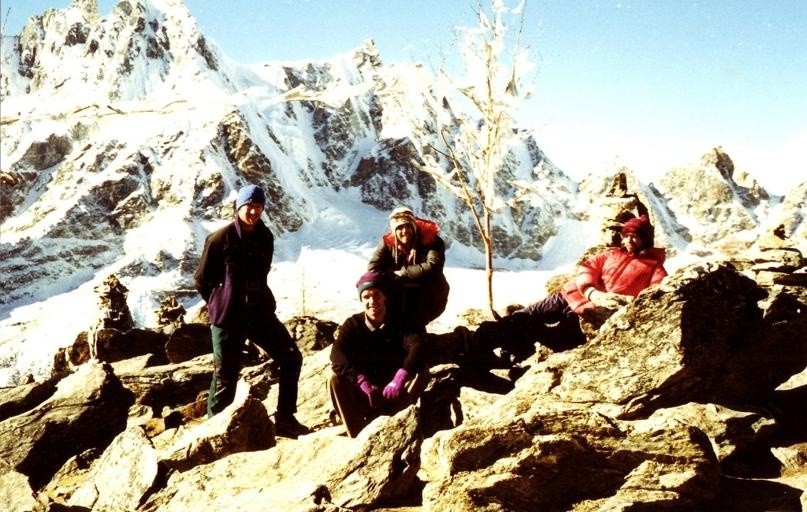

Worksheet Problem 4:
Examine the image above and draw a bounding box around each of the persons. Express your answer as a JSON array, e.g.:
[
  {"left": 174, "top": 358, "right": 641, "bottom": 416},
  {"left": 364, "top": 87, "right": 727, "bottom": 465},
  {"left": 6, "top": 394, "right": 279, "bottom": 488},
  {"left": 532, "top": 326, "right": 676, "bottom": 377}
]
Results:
[
  {"left": 367, "top": 204, "right": 451, "bottom": 333},
  {"left": 192, "top": 182, "right": 310, "bottom": 437},
  {"left": 328, "top": 273, "right": 429, "bottom": 437},
  {"left": 454, "top": 213, "right": 669, "bottom": 364}
]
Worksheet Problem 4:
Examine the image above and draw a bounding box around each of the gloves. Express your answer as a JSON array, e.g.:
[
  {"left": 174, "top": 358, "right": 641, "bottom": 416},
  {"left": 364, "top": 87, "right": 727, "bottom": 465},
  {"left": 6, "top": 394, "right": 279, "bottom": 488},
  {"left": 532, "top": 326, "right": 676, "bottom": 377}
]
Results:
[
  {"left": 589, "top": 289, "right": 636, "bottom": 312},
  {"left": 357, "top": 374, "right": 378, "bottom": 407},
  {"left": 382, "top": 368, "right": 408, "bottom": 399}
]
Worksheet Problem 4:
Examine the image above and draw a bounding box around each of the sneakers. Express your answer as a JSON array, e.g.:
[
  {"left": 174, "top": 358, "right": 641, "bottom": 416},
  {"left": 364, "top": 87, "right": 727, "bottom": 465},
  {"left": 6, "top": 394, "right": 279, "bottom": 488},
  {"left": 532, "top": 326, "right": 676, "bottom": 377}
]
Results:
[{"left": 274, "top": 412, "right": 308, "bottom": 432}]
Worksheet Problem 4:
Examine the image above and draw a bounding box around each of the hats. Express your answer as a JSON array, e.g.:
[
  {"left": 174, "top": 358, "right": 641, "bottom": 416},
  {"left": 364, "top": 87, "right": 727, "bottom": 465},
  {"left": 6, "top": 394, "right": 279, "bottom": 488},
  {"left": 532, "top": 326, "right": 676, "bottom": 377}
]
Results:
[
  {"left": 389, "top": 206, "right": 417, "bottom": 236},
  {"left": 622, "top": 214, "right": 649, "bottom": 238},
  {"left": 236, "top": 184, "right": 265, "bottom": 211},
  {"left": 356, "top": 271, "right": 379, "bottom": 301}
]
